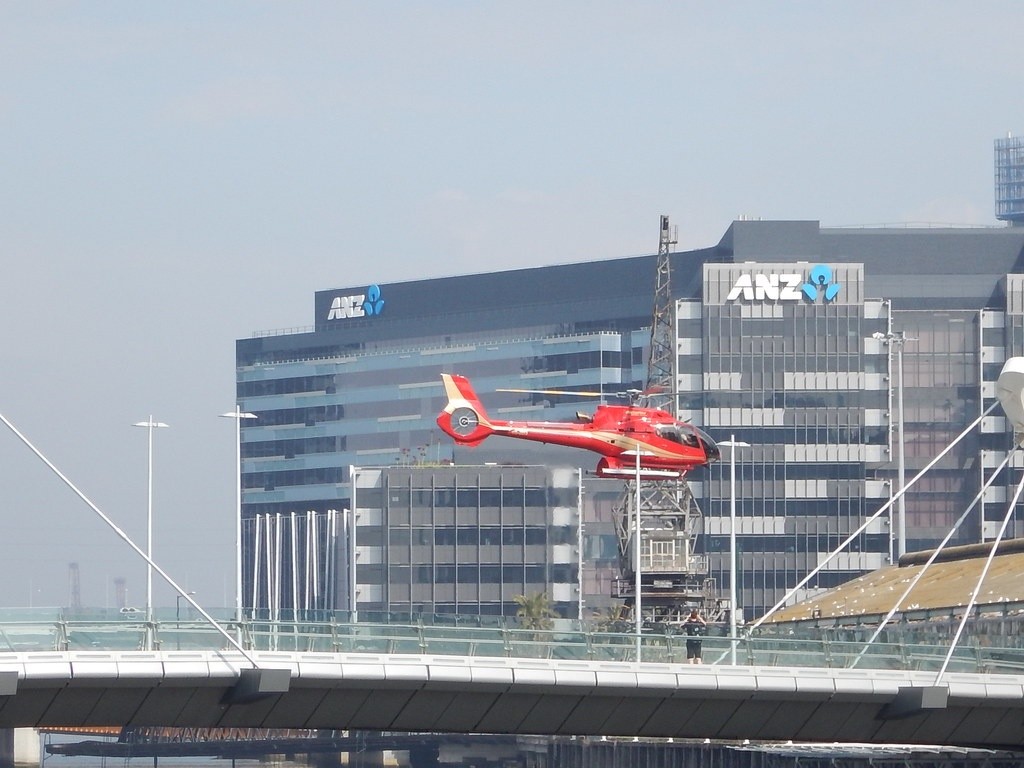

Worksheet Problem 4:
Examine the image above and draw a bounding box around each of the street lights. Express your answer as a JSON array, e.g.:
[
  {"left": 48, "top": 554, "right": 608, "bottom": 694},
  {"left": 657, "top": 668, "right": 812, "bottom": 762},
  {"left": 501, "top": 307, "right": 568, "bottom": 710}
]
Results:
[
  {"left": 620, "top": 444, "right": 654, "bottom": 664},
  {"left": 177, "top": 591, "right": 197, "bottom": 651},
  {"left": 132, "top": 414, "right": 170, "bottom": 650},
  {"left": 218, "top": 405, "right": 258, "bottom": 651},
  {"left": 714, "top": 434, "right": 751, "bottom": 665}
]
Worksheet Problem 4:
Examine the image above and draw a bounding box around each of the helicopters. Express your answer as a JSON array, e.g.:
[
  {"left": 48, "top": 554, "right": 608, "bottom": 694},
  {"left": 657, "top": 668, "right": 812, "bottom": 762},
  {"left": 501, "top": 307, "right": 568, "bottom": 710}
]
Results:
[{"left": 437, "top": 373, "right": 720, "bottom": 480}]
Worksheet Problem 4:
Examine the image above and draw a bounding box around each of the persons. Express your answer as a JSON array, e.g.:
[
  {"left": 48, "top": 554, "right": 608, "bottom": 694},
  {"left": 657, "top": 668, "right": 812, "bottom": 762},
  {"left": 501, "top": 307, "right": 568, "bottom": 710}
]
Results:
[
  {"left": 680, "top": 430, "right": 697, "bottom": 446},
  {"left": 681, "top": 610, "right": 708, "bottom": 664}
]
